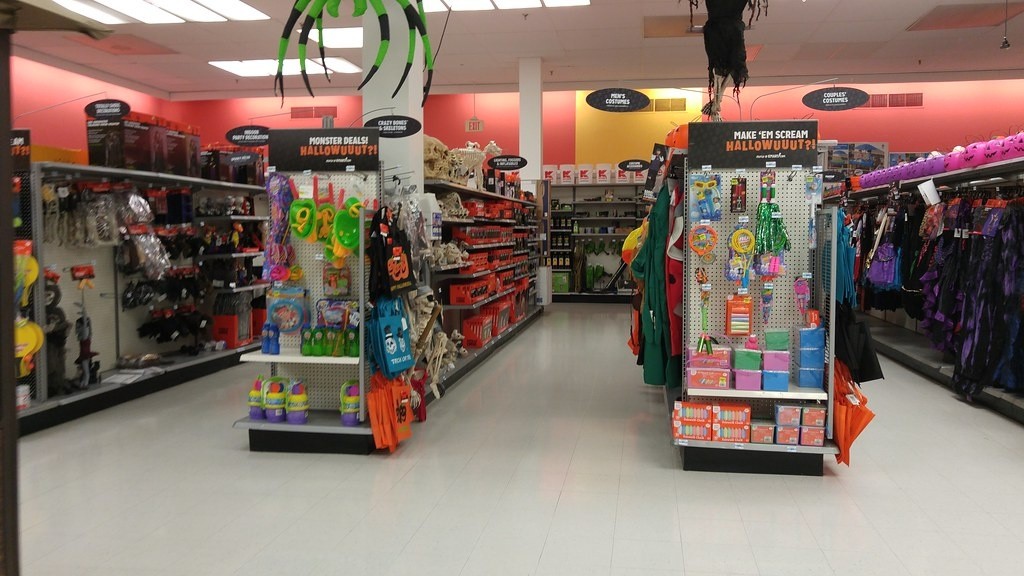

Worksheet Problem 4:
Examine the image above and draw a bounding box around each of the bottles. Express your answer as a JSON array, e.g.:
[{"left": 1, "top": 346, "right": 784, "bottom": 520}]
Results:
[
  {"left": 557, "top": 233, "right": 563, "bottom": 249},
  {"left": 559, "top": 252, "right": 564, "bottom": 269},
  {"left": 551, "top": 233, "right": 557, "bottom": 249},
  {"left": 553, "top": 252, "right": 557, "bottom": 268},
  {"left": 542, "top": 251, "right": 549, "bottom": 266},
  {"left": 555, "top": 212, "right": 560, "bottom": 229},
  {"left": 573, "top": 221, "right": 578, "bottom": 234},
  {"left": 564, "top": 233, "right": 570, "bottom": 249},
  {"left": 565, "top": 252, "right": 570, "bottom": 268},
  {"left": 550, "top": 216, "right": 555, "bottom": 229},
  {"left": 561, "top": 212, "right": 565, "bottom": 229},
  {"left": 567, "top": 212, "right": 572, "bottom": 229}
]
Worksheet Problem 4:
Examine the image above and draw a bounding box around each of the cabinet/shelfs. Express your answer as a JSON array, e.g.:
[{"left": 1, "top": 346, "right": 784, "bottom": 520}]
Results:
[{"left": 0, "top": 120, "right": 1024, "bottom": 477}]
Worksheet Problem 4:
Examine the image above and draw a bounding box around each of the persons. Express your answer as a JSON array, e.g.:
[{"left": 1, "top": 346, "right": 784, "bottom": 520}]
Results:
[
  {"left": 258, "top": 157, "right": 264, "bottom": 185},
  {"left": 154, "top": 132, "right": 170, "bottom": 173},
  {"left": 189, "top": 140, "right": 199, "bottom": 175},
  {"left": 104, "top": 131, "right": 121, "bottom": 166}
]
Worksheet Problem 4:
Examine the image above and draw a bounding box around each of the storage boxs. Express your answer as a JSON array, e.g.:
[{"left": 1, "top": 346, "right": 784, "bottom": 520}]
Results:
[
  {"left": 200, "top": 140, "right": 270, "bottom": 186},
  {"left": 462, "top": 314, "right": 494, "bottom": 348},
  {"left": 459, "top": 252, "right": 490, "bottom": 275},
  {"left": 552, "top": 273, "right": 572, "bottom": 293},
  {"left": 84, "top": 98, "right": 203, "bottom": 177},
  {"left": 450, "top": 280, "right": 489, "bottom": 306},
  {"left": 452, "top": 226, "right": 486, "bottom": 245},
  {"left": 481, "top": 301, "right": 512, "bottom": 337},
  {"left": 529, "top": 265, "right": 552, "bottom": 306}
]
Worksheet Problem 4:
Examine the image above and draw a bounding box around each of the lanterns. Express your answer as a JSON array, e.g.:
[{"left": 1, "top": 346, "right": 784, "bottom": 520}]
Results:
[{"left": 859, "top": 132, "right": 1024, "bottom": 189}]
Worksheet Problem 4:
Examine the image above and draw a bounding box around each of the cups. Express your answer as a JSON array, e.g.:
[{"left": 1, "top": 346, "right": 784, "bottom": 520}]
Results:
[
  {"left": 586, "top": 228, "right": 593, "bottom": 234},
  {"left": 600, "top": 227, "right": 607, "bottom": 234},
  {"left": 595, "top": 228, "right": 600, "bottom": 234},
  {"left": 608, "top": 227, "right": 614, "bottom": 233},
  {"left": 579, "top": 227, "right": 585, "bottom": 233}
]
